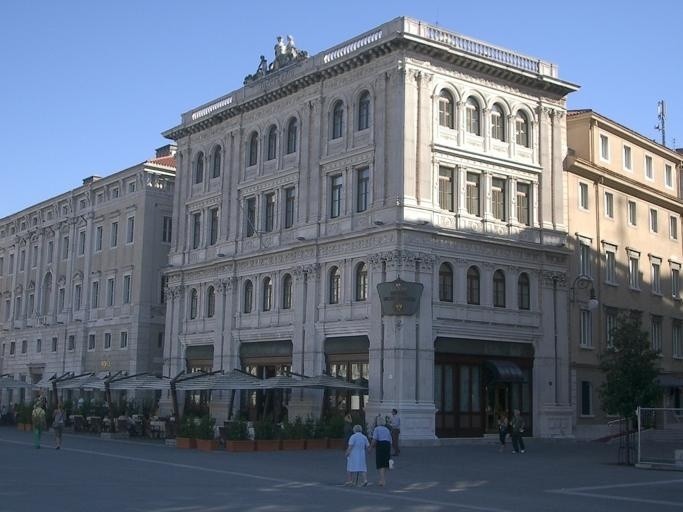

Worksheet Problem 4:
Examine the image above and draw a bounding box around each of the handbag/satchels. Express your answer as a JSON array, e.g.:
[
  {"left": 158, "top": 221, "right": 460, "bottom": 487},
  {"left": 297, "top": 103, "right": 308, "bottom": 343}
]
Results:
[
  {"left": 51, "top": 421, "right": 59, "bottom": 428},
  {"left": 388, "top": 460, "right": 393, "bottom": 470}
]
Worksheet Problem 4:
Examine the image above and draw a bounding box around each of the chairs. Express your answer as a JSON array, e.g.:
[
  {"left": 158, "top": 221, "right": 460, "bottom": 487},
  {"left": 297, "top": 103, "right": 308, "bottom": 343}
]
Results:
[{"left": 69, "top": 413, "right": 172, "bottom": 439}]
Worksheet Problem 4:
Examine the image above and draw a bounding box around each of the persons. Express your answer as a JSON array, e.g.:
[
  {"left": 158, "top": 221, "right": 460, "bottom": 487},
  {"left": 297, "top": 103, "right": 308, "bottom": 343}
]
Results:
[
  {"left": 386, "top": 408, "right": 401, "bottom": 456},
  {"left": 256, "top": 55, "right": 267, "bottom": 76},
  {"left": 507, "top": 409, "right": 527, "bottom": 453},
  {"left": 495, "top": 411, "right": 509, "bottom": 449},
  {"left": 366, "top": 415, "right": 392, "bottom": 485},
  {"left": 343, "top": 424, "right": 370, "bottom": 486},
  {"left": 30, "top": 400, "right": 45, "bottom": 449},
  {"left": 52, "top": 401, "right": 64, "bottom": 449},
  {"left": 343, "top": 413, "right": 352, "bottom": 448},
  {"left": 484, "top": 400, "right": 510, "bottom": 433},
  {"left": 273, "top": 36, "right": 285, "bottom": 57},
  {"left": 285, "top": 34, "right": 296, "bottom": 60}
]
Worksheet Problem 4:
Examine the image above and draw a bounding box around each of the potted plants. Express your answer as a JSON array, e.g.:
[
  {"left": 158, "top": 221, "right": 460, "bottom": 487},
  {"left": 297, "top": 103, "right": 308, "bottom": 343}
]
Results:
[
  {"left": 16, "top": 402, "right": 34, "bottom": 433},
  {"left": 172, "top": 413, "right": 350, "bottom": 452}
]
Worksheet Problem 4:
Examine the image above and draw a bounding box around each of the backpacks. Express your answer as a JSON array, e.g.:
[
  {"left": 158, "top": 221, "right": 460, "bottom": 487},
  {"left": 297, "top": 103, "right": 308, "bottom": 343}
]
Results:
[{"left": 32, "top": 409, "right": 42, "bottom": 427}]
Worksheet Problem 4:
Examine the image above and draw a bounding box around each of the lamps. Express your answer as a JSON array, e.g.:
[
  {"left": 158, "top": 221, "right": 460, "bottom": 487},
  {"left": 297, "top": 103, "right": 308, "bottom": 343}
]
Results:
[{"left": 570, "top": 273, "right": 599, "bottom": 310}]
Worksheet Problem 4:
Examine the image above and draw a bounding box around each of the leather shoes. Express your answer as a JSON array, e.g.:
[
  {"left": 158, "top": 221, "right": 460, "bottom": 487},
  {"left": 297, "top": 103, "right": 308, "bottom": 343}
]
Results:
[
  {"left": 344, "top": 481, "right": 352, "bottom": 486},
  {"left": 360, "top": 480, "right": 367, "bottom": 486}
]
